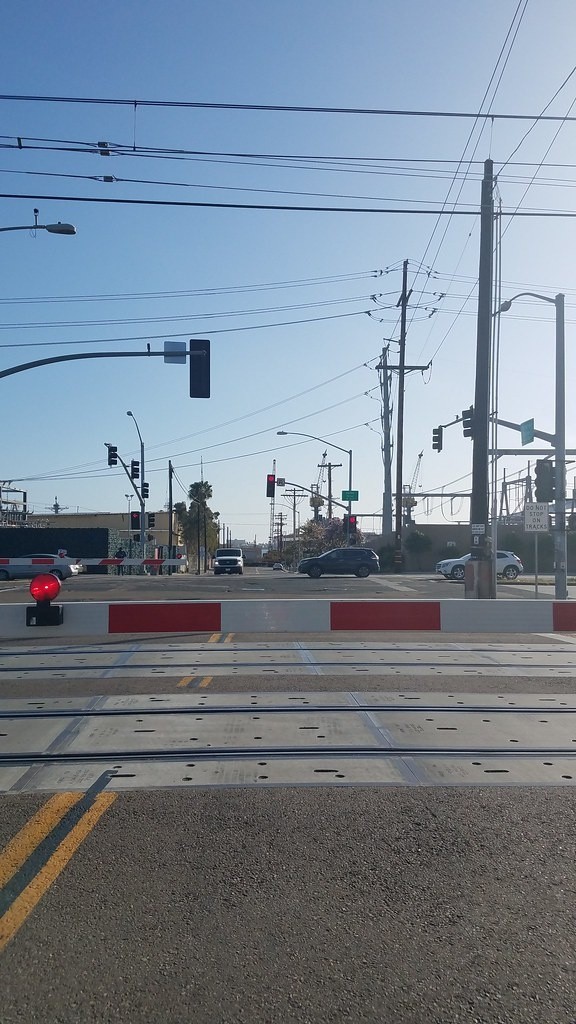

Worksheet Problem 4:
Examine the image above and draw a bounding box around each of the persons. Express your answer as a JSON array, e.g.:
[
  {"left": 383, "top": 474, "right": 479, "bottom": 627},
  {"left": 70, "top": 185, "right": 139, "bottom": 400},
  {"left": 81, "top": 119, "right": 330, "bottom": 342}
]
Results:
[{"left": 114, "top": 547, "right": 127, "bottom": 576}]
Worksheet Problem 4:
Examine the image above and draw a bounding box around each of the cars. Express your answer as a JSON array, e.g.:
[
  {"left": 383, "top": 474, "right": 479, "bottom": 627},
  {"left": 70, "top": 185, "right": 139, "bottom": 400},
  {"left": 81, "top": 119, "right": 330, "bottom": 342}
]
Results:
[
  {"left": 0, "top": 554, "right": 84, "bottom": 581},
  {"left": 298, "top": 547, "right": 380, "bottom": 578},
  {"left": 211, "top": 548, "right": 246, "bottom": 575},
  {"left": 273, "top": 562, "right": 283, "bottom": 570},
  {"left": 436, "top": 550, "right": 523, "bottom": 580}
]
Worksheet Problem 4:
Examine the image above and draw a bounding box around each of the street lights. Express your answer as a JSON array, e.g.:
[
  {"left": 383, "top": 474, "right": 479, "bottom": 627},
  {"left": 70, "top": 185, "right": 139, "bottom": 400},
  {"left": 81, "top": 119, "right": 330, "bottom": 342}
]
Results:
[
  {"left": 498, "top": 293, "right": 569, "bottom": 601},
  {"left": 277, "top": 431, "right": 352, "bottom": 547},
  {"left": 127, "top": 411, "right": 150, "bottom": 575},
  {"left": 125, "top": 494, "right": 134, "bottom": 575},
  {"left": 270, "top": 503, "right": 300, "bottom": 563}
]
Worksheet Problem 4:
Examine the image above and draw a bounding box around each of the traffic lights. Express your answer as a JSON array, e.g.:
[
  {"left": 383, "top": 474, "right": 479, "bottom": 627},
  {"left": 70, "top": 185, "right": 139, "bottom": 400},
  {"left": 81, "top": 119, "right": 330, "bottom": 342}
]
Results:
[
  {"left": 108, "top": 446, "right": 118, "bottom": 465},
  {"left": 535, "top": 459, "right": 556, "bottom": 503},
  {"left": 131, "top": 511, "right": 140, "bottom": 530},
  {"left": 134, "top": 534, "right": 154, "bottom": 542},
  {"left": 148, "top": 514, "right": 155, "bottom": 528},
  {"left": 267, "top": 474, "right": 275, "bottom": 497},
  {"left": 349, "top": 516, "right": 356, "bottom": 533},
  {"left": 142, "top": 483, "right": 149, "bottom": 498},
  {"left": 131, "top": 461, "right": 139, "bottom": 479},
  {"left": 432, "top": 405, "right": 473, "bottom": 453}
]
